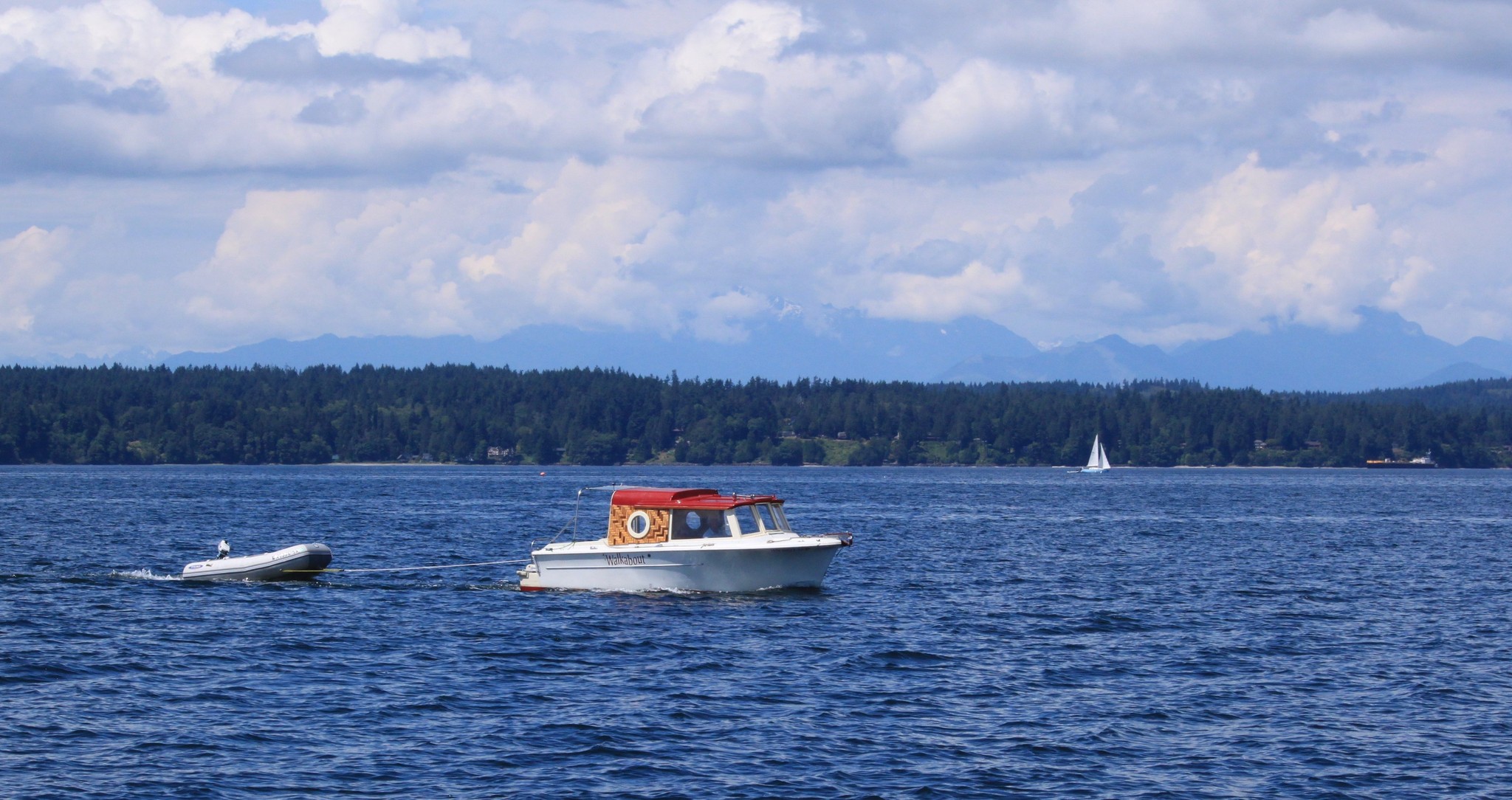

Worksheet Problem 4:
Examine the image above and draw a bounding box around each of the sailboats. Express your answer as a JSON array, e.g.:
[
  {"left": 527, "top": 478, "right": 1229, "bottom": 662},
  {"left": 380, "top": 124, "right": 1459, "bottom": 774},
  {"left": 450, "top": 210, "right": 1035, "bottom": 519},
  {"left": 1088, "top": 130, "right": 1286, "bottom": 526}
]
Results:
[{"left": 1075, "top": 433, "right": 1112, "bottom": 474}]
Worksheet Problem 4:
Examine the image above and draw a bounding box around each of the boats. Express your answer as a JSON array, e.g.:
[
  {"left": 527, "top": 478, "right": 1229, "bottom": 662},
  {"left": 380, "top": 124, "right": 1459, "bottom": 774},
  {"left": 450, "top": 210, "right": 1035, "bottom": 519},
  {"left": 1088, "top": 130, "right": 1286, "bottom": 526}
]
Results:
[
  {"left": 517, "top": 483, "right": 853, "bottom": 597},
  {"left": 1365, "top": 448, "right": 1438, "bottom": 469},
  {"left": 181, "top": 539, "right": 333, "bottom": 582}
]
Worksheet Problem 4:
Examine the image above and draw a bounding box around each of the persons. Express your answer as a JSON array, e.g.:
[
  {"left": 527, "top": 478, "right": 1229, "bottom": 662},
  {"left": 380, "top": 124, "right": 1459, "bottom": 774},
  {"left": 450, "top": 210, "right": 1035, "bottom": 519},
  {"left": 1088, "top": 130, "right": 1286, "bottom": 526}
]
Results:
[{"left": 703, "top": 513, "right": 733, "bottom": 538}]
